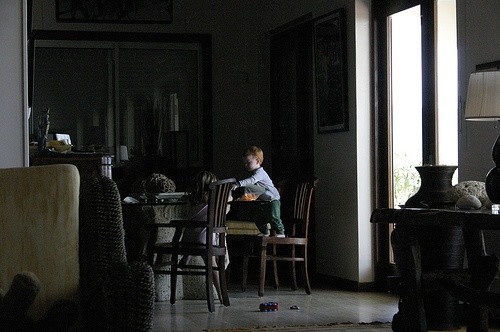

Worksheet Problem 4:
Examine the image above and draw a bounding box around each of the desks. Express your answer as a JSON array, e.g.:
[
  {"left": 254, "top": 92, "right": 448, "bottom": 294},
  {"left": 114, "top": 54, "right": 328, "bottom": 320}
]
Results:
[
  {"left": 123, "top": 200, "right": 276, "bottom": 297},
  {"left": 369, "top": 207, "right": 500, "bottom": 332}
]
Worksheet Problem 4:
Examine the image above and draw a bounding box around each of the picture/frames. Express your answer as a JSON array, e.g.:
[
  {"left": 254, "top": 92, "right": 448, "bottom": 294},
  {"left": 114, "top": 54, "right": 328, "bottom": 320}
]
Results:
[
  {"left": 312, "top": 6, "right": 350, "bottom": 134},
  {"left": 55, "top": 0, "right": 173, "bottom": 24}
]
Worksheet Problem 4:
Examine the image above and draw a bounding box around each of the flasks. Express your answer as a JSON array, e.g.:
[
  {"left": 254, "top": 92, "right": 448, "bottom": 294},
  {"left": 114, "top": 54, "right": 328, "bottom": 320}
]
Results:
[{"left": 101, "top": 157, "right": 113, "bottom": 180}]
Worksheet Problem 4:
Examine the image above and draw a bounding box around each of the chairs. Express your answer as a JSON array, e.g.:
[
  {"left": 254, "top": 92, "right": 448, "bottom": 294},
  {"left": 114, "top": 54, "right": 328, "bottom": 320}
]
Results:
[
  {"left": 241, "top": 176, "right": 321, "bottom": 296},
  {"left": 143, "top": 177, "right": 237, "bottom": 313}
]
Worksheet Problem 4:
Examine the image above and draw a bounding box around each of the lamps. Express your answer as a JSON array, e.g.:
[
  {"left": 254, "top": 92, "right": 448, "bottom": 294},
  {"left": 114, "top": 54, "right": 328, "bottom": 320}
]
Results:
[{"left": 462, "top": 60, "right": 500, "bottom": 211}]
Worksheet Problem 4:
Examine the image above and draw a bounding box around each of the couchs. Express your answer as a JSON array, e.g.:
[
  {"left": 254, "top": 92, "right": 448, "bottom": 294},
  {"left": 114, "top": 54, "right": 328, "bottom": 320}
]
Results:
[{"left": 0, "top": 163, "right": 156, "bottom": 332}]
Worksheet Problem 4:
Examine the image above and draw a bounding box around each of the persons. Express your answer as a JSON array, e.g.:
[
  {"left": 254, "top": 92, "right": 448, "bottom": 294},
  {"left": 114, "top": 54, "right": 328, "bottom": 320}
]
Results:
[
  {"left": 230, "top": 146, "right": 285, "bottom": 237},
  {"left": 179, "top": 171, "right": 230, "bottom": 304}
]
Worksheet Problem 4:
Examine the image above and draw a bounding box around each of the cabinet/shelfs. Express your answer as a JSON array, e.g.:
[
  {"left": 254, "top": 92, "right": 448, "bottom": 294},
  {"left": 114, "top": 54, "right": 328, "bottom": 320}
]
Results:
[{"left": 35, "top": 153, "right": 165, "bottom": 197}]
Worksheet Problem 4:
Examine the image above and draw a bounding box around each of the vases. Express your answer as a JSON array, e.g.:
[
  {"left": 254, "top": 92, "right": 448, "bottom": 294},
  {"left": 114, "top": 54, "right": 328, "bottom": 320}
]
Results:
[{"left": 390, "top": 164, "right": 465, "bottom": 332}]
